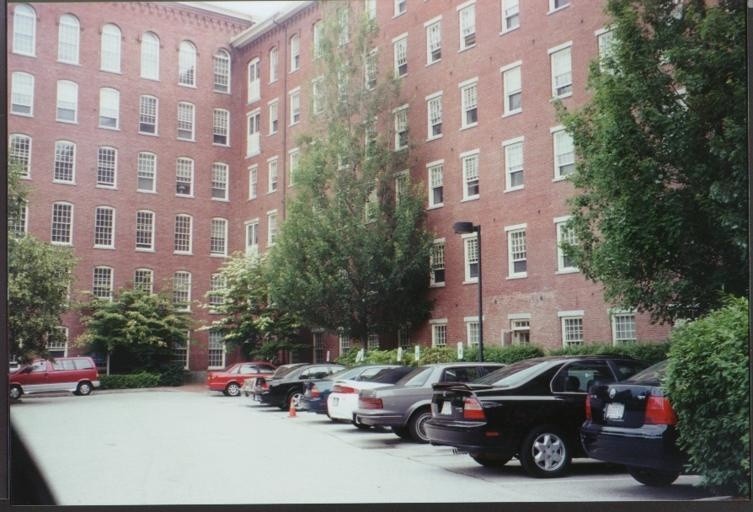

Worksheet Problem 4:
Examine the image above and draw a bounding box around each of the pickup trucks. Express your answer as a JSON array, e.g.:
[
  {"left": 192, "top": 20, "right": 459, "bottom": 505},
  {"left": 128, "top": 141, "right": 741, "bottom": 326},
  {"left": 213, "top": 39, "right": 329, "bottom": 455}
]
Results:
[{"left": 240, "top": 364, "right": 308, "bottom": 404}]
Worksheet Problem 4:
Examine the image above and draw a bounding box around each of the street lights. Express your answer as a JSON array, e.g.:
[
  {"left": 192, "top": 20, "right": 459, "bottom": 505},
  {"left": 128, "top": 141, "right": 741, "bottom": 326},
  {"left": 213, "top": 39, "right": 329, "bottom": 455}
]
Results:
[{"left": 454, "top": 222, "right": 484, "bottom": 362}]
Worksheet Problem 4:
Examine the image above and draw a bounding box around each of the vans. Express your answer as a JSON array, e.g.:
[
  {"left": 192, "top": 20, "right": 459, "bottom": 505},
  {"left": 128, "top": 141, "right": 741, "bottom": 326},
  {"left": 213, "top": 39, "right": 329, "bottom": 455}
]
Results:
[{"left": 7, "top": 356, "right": 101, "bottom": 399}]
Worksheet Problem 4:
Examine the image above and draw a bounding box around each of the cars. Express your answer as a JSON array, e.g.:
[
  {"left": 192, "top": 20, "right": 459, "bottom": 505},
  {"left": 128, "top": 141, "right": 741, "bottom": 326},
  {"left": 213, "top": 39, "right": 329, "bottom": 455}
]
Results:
[
  {"left": 423, "top": 354, "right": 655, "bottom": 479},
  {"left": 579, "top": 357, "right": 706, "bottom": 487},
  {"left": 357, "top": 362, "right": 510, "bottom": 444},
  {"left": 300, "top": 364, "right": 375, "bottom": 419},
  {"left": 206, "top": 362, "right": 277, "bottom": 398},
  {"left": 253, "top": 363, "right": 347, "bottom": 412},
  {"left": 326, "top": 365, "right": 424, "bottom": 433}
]
